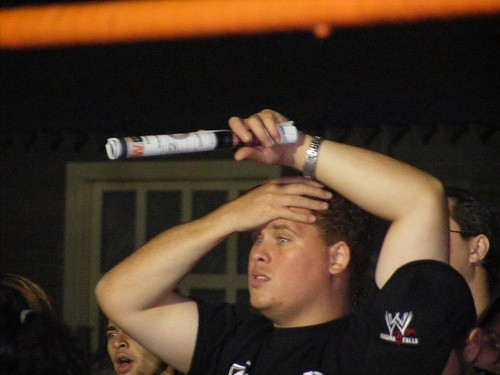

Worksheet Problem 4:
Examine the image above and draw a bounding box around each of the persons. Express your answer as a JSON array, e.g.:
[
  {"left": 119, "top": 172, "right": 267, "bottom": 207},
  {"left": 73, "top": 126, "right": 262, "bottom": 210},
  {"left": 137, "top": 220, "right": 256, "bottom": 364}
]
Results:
[
  {"left": 442, "top": 181, "right": 500, "bottom": 375},
  {"left": 0, "top": 274, "right": 183, "bottom": 375},
  {"left": 96, "top": 109, "right": 478, "bottom": 375}
]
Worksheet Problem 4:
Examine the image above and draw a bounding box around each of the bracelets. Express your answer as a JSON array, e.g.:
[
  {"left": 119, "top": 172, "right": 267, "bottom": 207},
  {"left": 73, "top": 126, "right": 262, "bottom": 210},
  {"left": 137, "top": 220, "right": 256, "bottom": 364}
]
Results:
[{"left": 299, "top": 135, "right": 323, "bottom": 179}]
